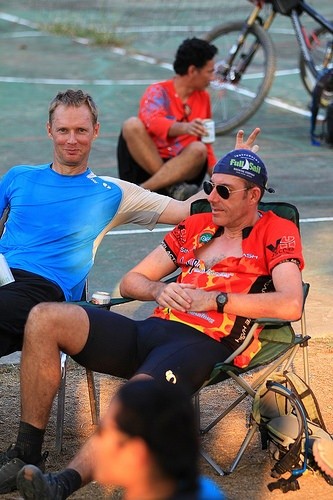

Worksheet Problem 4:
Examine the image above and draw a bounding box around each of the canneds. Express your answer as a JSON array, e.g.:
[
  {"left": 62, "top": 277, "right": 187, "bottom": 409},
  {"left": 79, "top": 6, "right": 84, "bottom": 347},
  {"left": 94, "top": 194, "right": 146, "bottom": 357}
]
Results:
[
  {"left": 201, "top": 118, "right": 216, "bottom": 143},
  {"left": 91, "top": 290, "right": 111, "bottom": 311}
]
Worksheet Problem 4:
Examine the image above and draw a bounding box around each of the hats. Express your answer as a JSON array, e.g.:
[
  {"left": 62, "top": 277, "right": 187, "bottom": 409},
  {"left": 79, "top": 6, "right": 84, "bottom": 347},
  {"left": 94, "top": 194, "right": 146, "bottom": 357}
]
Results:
[{"left": 213, "top": 148, "right": 275, "bottom": 193}]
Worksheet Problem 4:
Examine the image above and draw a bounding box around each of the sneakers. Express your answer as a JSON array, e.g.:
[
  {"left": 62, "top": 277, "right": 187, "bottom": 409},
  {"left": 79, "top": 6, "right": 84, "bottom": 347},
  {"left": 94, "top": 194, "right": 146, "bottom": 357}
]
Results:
[
  {"left": 0, "top": 442, "right": 50, "bottom": 495},
  {"left": 16, "top": 464, "right": 70, "bottom": 500},
  {"left": 168, "top": 181, "right": 199, "bottom": 201}
]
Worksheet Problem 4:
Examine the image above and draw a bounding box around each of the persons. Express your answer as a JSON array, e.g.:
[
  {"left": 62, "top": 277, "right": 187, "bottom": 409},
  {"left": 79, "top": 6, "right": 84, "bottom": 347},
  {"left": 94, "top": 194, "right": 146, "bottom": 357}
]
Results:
[
  {"left": 116, "top": 36, "right": 218, "bottom": 201},
  {"left": 89, "top": 381, "right": 228, "bottom": 500},
  {"left": 0, "top": 88, "right": 261, "bottom": 358},
  {"left": 0, "top": 149, "right": 305, "bottom": 500}
]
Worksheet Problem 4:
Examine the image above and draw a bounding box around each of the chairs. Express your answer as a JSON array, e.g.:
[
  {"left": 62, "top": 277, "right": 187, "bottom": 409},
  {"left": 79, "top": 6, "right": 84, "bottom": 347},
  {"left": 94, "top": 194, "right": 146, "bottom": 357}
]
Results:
[{"left": 55, "top": 199, "right": 312, "bottom": 477}]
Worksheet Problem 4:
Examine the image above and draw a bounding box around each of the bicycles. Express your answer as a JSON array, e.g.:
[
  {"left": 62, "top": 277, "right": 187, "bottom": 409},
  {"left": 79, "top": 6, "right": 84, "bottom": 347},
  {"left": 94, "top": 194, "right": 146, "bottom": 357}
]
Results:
[{"left": 197, "top": 0, "right": 333, "bottom": 149}]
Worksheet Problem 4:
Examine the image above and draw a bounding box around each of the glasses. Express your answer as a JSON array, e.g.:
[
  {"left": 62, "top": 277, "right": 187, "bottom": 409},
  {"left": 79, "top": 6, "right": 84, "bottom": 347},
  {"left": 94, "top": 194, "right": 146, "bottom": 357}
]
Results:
[{"left": 202, "top": 180, "right": 251, "bottom": 199}]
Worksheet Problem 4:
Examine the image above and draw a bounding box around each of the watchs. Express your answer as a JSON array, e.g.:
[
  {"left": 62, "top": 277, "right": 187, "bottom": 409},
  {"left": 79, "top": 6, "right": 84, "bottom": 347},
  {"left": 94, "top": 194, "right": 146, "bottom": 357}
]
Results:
[{"left": 216, "top": 292, "right": 228, "bottom": 314}]
